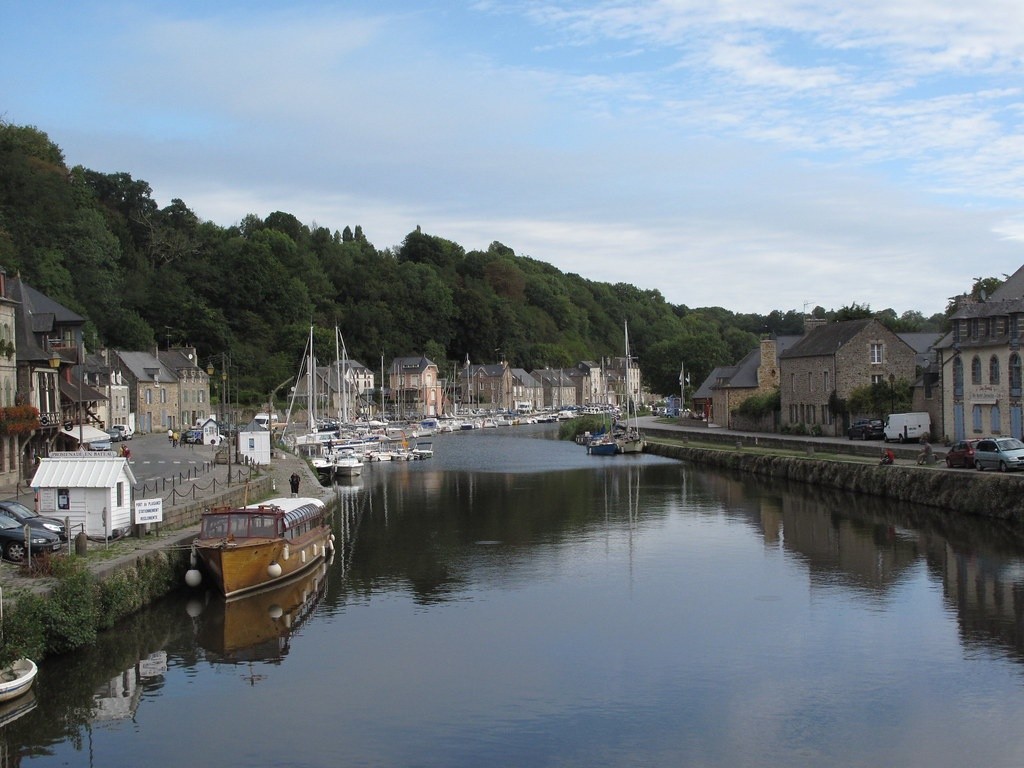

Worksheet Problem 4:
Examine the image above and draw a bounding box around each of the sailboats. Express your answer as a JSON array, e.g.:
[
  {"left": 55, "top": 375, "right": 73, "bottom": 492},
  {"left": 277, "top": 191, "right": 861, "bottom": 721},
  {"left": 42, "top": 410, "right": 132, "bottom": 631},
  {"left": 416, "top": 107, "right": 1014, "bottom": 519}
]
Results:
[{"left": 282, "top": 322, "right": 643, "bottom": 485}]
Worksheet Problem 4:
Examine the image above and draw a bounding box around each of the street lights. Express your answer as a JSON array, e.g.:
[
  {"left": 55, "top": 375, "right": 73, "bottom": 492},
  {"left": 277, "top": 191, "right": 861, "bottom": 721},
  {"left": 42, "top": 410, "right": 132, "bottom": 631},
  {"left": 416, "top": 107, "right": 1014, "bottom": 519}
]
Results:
[
  {"left": 208, "top": 353, "right": 228, "bottom": 427},
  {"left": 49, "top": 338, "right": 83, "bottom": 449}
]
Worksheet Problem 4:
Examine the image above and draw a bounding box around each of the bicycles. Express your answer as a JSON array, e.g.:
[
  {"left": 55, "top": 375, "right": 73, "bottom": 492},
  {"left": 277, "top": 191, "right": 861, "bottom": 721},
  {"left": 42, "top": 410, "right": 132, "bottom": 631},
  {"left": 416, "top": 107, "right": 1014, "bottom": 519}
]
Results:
[{"left": 173, "top": 439, "right": 177, "bottom": 448}]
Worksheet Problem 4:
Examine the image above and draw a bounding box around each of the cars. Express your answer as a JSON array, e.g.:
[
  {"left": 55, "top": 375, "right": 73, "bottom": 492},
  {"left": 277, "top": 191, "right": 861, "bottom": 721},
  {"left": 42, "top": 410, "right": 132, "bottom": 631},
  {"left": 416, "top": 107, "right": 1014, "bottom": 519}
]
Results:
[
  {"left": 946, "top": 438, "right": 982, "bottom": 468},
  {"left": 186, "top": 430, "right": 226, "bottom": 444},
  {"left": 112, "top": 424, "right": 133, "bottom": 440},
  {"left": 974, "top": 435, "right": 1024, "bottom": 471},
  {"left": 0, "top": 512, "right": 60, "bottom": 563},
  {"left": 89, "top": 440, "right": 112, "bottom": 451},
  {"left": 847, "top": 419, "right": 885, "bottom": 441},
  {"left": 0, "top": 498, "right": 68, "bottom": 541},
  {"left": 254, "top": 413, "right": 279, "bottom": 431},
  {"left": 105, "top": 428, "right": 123, "bottom": 442}
]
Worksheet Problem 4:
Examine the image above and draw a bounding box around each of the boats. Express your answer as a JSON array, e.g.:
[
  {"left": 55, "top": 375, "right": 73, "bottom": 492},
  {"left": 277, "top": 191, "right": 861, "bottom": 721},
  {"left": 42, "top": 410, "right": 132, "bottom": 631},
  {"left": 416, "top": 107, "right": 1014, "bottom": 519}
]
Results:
[
  {"left": 0, "top": 658, "right": 39, "bottom": 704},
  {"left": 189, "top": 495, "right": 332, "bottom": 598}
]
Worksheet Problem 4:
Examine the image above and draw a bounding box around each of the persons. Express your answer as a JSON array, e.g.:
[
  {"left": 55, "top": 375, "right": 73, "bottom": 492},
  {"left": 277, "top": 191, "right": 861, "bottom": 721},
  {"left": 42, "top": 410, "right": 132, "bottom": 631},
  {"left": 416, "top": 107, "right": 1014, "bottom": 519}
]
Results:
[
  {"left": 289, "top": 471, "right": 301, "bottom": 498},
  {"left": 172, "top": 432, "right": 179, "bottom": 447},
  {"left": 123, "top": 446, "right": 132, "bottom": 463},
  {"left": 168, "top": 429, "right": 173, "bottom": 440},
  {"left": 916, "top": 439, "right": 933, "bottom": 466},
  {"left": 118, "top": 444, "right": 126, "bottom": 457},
  {"left": 326, "top": 439, "right": 334, "bottom": 454}
]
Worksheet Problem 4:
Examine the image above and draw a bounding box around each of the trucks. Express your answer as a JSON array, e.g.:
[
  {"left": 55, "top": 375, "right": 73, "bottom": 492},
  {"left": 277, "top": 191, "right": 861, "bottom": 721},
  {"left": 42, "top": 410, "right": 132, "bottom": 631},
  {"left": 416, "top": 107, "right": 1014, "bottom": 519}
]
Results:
[{"left": 883, "top": 412, "right": 932, "bottom": 443}]
